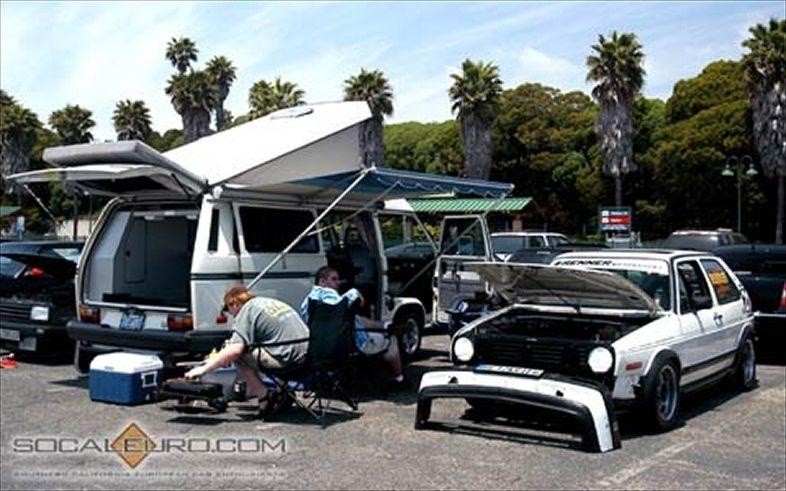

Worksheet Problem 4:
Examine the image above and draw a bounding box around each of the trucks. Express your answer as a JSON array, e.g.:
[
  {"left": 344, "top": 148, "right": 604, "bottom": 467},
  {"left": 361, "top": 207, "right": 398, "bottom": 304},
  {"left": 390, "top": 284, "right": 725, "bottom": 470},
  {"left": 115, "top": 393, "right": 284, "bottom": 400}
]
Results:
[{"left": 5, "top": 99, "right": 495, "bottom": 376}]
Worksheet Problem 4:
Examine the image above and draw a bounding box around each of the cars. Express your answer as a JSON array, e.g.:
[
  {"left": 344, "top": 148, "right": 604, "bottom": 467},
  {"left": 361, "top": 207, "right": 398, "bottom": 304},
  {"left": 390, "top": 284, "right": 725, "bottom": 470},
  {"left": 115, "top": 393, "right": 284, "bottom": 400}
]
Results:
[
  {"left": 444, "top": 242, "right": 759, "bottom": 430},
  {"left": 0, "top": 235, "right": 87, "bottom": 363}
]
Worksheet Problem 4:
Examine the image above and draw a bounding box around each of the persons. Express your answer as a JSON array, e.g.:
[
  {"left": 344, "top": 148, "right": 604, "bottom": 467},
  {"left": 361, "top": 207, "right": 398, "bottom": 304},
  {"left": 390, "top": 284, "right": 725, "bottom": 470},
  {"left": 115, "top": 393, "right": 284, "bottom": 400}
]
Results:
[
  {"left": 301, "top": 264, "right": 411, "bottom": 394},
  {"left": 183, "top": 284, "right": 310, "bottom": 421}
]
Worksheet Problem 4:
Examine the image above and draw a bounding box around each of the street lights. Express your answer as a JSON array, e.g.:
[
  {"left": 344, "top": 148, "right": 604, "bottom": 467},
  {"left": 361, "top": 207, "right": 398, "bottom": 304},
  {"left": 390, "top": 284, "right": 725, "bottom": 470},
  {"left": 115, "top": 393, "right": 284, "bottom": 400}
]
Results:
[{"left": 720, "top": 152, "right": 760, "bottom": 231}]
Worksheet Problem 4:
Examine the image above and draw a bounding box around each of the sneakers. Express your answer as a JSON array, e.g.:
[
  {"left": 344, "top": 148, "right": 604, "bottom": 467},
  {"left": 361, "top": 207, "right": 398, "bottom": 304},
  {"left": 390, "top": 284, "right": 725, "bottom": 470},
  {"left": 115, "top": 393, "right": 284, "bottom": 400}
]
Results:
[{"left": 259, "top": 389, "right": 279, "bottom": 418}]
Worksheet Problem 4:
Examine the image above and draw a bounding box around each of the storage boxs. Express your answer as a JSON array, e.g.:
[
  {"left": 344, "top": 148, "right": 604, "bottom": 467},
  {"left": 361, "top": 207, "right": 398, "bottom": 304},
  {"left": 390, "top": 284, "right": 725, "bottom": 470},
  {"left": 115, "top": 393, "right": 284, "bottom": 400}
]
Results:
[
  {"left": 89, "top": 352, "right": 164, "bottom": 405},
  {"left": 202, "top": 366, "right": 237, "bottom": 404}
]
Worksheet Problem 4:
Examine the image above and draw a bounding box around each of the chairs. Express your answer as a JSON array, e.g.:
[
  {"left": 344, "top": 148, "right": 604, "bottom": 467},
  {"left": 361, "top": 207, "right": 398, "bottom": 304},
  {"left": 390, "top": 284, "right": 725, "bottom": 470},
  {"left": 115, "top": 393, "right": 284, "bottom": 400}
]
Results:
[{"left": 263, "top": 297, "right": 360, "bottom": 423}]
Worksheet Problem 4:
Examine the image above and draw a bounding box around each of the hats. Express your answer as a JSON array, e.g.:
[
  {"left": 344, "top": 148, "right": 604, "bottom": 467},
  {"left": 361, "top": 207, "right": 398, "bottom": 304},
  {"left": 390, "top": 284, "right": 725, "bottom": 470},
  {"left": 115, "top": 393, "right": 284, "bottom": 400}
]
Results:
[{"left": 222, "top": 285, "right": 252, "bottom": 310}]
